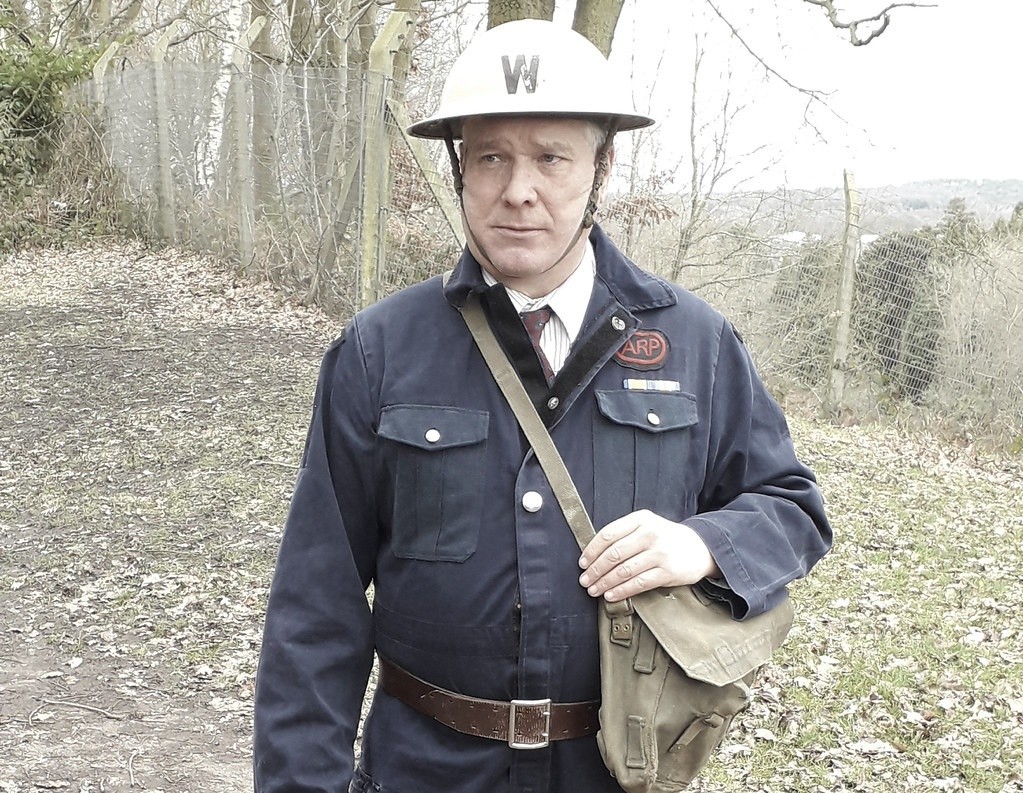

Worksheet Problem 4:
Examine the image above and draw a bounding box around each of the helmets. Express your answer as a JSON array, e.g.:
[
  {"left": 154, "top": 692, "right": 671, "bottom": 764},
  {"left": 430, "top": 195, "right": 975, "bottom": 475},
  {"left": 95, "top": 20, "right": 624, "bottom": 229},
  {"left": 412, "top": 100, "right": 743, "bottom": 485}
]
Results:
[{"left": 404, "top": 17, "right": 659, "bottom": 140}]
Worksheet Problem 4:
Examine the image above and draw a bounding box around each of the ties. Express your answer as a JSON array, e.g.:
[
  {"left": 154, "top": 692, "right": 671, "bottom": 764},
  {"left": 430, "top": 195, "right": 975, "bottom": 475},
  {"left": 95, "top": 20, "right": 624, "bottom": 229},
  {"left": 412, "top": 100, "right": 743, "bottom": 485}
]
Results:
[{"left": 519, "top": 304, "right": 557, "bottom": 393}]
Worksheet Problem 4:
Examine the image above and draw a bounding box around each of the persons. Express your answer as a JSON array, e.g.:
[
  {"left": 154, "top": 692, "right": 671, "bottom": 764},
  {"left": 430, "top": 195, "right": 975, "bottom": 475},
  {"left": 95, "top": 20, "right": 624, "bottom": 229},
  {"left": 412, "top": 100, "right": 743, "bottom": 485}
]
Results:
[{"left": 253, "top": 19, "right": 832, "bottom": 793}]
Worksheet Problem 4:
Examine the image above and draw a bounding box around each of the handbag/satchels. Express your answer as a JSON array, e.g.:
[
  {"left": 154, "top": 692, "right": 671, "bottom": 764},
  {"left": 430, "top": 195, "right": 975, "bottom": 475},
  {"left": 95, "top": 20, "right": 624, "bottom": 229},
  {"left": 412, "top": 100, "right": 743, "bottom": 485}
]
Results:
[{"left": 442, "top": 267, "right": 794, "bottom": 793}]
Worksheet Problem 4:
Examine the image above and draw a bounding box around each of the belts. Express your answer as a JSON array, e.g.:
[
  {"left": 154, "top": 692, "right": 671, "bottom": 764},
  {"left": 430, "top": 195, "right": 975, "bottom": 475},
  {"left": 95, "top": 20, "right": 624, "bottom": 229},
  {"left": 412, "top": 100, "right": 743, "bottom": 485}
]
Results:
[{"left": 376, "top": 649, "right": 601, "bottom": 751}]
